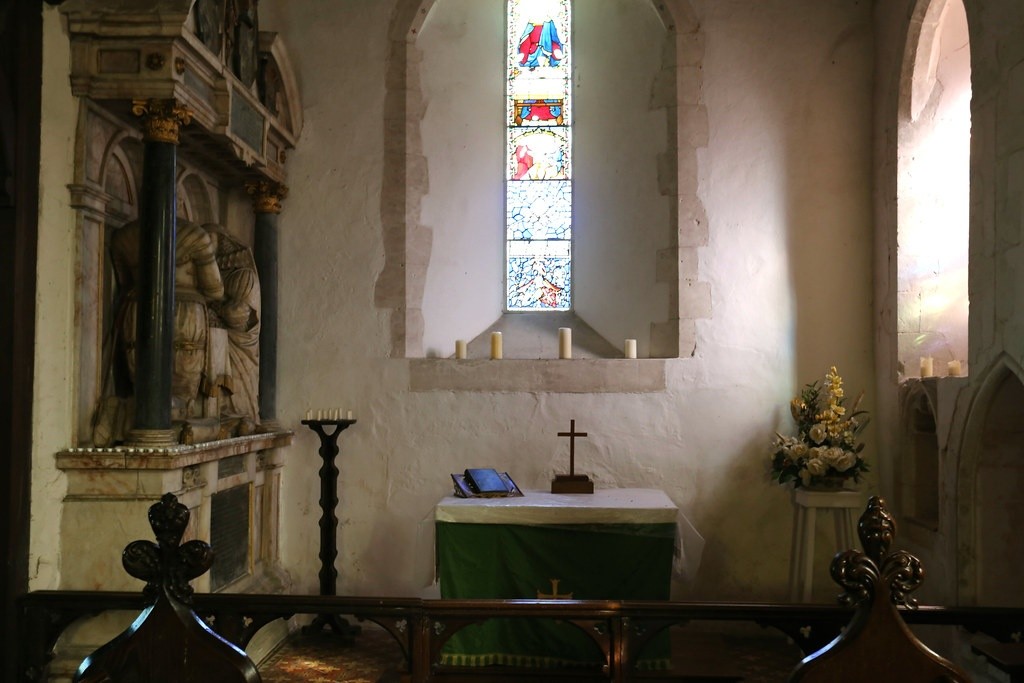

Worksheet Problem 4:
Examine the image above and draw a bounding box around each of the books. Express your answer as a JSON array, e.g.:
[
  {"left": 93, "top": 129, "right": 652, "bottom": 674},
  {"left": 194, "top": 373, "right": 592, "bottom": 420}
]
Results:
[{"left": 464, "top": 468, "right": 509, "bottom": 497}]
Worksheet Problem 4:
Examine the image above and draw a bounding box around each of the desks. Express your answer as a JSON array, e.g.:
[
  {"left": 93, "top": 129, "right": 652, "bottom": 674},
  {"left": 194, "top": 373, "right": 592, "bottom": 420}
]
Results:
[{"left": 432, "top": 488, "right": 680, "bottom": 673}]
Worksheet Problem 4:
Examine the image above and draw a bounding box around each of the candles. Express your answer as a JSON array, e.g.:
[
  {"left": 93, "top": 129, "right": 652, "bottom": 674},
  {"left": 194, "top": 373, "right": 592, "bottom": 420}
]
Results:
[
  {"left": 559, "top": 328, "right": 573, "bottom": 359},
  {"left": 946, "top": 359, "right": 962, "bottom": 378},
  {"left": 624, "top": 339, "right": 637, "bottom": 360},
  {"left": 491, "top": 331, "right": 502, "bottom": 360},
  {"left": 306, "top": 407, "right": 354, "bottom": 420},
  {"left": 921, "top": 355, "right": 934, "bottom": 378},
  {"left": 455, "top": 339, "right": 466, "bottom": 361}
]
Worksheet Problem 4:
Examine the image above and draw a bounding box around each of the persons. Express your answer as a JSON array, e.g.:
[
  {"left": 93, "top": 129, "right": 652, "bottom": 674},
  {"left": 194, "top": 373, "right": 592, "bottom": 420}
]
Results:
[{"left": 93, "top": 182, "right": 261, "bottom": 447}]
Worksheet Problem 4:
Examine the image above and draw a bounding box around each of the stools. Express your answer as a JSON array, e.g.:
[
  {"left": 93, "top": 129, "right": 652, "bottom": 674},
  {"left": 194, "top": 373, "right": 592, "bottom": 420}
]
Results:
[{"left": 789, "top": 492, "right": 861, "bottom": 604}]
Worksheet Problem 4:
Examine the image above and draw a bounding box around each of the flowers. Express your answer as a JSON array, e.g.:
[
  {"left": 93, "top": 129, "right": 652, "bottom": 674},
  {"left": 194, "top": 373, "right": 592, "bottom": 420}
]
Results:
[{"left": 770, "top": 365, "right": 872, "bottom": 484}]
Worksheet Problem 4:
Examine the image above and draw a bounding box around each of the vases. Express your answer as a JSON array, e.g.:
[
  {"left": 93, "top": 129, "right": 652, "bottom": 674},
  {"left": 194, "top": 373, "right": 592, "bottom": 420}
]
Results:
[{"left": 807, "top": 475, "right": 843, "bottom": 491}]
